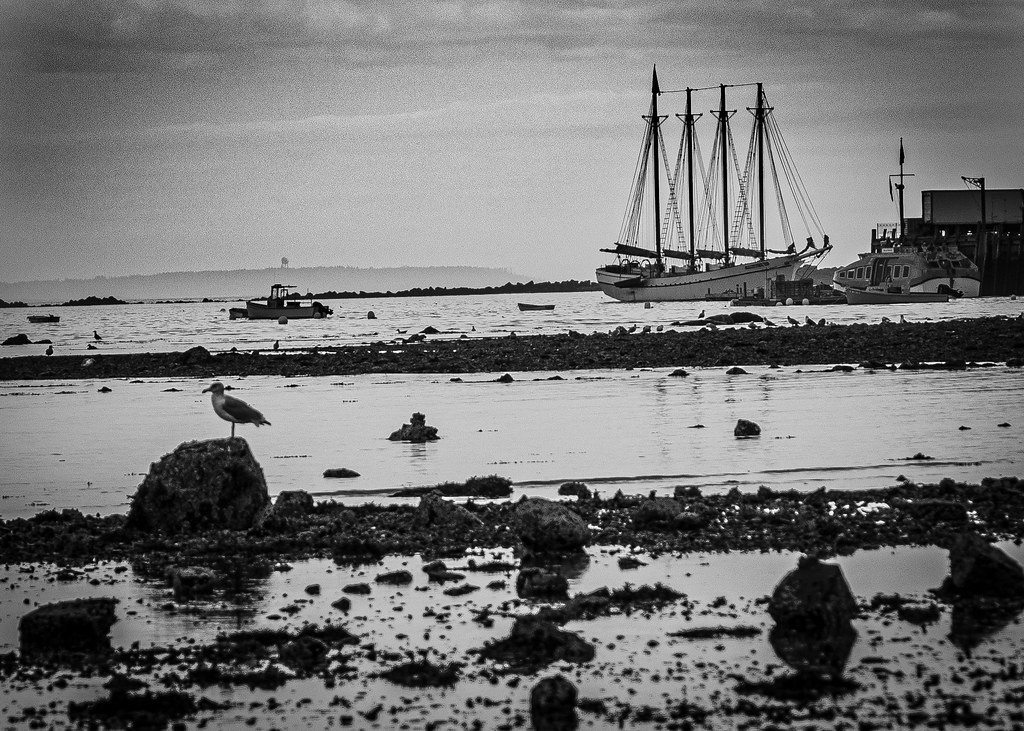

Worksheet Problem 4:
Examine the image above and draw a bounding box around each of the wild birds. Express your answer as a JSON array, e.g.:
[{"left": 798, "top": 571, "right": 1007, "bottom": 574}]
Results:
[
  {"left": 203, "top": 382, "right": 272, "bottom": 438},
  {"left": 271, "top": 326, "right": 480, "bottom": 352},
  {"left": 39, "top": 325, "right": 108, "bottom": 359},
  {"left": 627, "top": 309, "right": 907, "bottom": 337}
]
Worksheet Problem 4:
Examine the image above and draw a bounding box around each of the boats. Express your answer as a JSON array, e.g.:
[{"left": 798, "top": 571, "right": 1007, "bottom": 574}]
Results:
[
  {"left": 518, "top": 303, "right": 556, "bottom": 310},
  {"left": 247, "top": 284, "right": 330, "bottom": 320},
  {"left": 705, "top": 286, "right": 755, "bottom": 300},
  {"left": 845, "top": 287, "right": 947, "bottom": 305},
  {"left": 838, "top": 249, "right": 982, "bottom": 299},
  {"left": 28, "top": 314, "right": 61, "bottom": 322}
]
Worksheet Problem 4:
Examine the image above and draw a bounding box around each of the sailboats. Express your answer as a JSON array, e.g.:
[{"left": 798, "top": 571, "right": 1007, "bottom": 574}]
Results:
[{"left": 597, "top": 62, "right": 833, "bottom": 302}]
